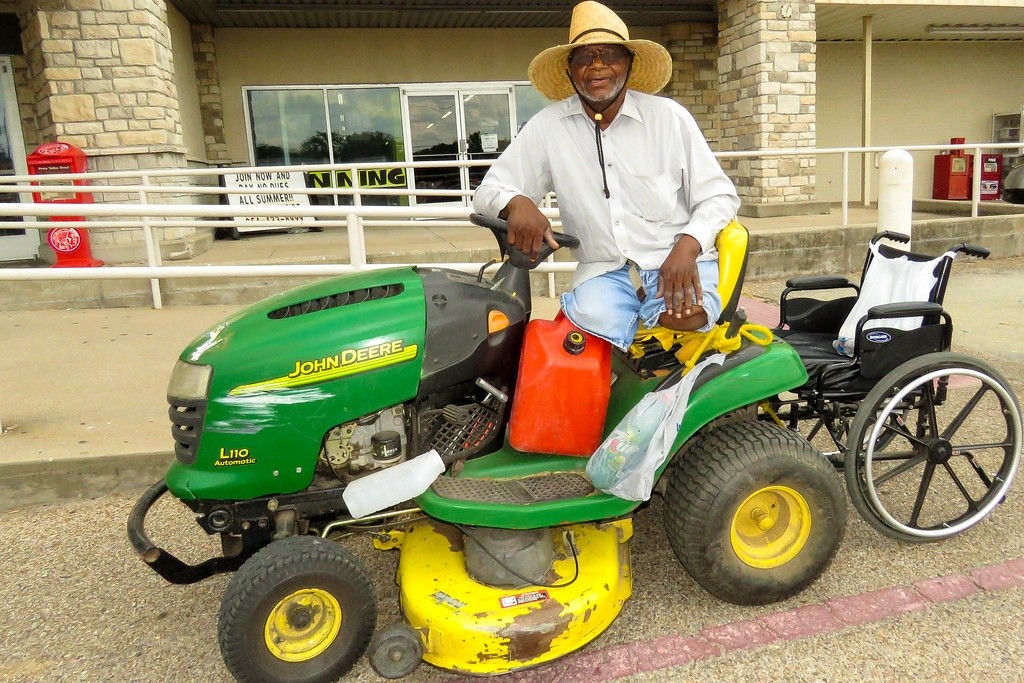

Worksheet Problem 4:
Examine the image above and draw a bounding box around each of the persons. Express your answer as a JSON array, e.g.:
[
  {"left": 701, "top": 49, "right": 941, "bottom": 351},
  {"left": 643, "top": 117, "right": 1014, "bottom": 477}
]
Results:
[{"left": 474, "top": 1, "right": 742, "bottom": 352}]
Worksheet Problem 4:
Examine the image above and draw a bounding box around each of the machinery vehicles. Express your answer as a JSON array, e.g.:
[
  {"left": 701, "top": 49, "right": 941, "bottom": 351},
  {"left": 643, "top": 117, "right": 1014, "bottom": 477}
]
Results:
[{"left": 126, "top": 209, "right": 848, "bottom": 682}]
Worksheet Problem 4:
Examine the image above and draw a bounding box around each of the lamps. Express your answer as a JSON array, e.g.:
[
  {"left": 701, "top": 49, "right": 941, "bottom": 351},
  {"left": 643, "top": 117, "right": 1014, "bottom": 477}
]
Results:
[{"left": 928, "top": 26, "right": 1024, "bottom": 35}]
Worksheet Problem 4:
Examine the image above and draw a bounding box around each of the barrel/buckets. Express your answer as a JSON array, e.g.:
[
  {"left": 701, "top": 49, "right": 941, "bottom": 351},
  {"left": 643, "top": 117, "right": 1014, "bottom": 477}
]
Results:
[{"left": 508, "top": 307, "right": 611, "bottom": 459}]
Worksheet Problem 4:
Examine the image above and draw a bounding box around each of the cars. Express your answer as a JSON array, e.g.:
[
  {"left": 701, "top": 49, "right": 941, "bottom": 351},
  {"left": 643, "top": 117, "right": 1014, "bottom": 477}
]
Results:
[{"left": 425, "top": 180, "right": 480, "bottom": 203}]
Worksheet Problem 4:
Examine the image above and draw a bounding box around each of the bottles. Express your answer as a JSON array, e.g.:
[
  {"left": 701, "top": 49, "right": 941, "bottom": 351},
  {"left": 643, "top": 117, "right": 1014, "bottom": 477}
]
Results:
[{"left": 833, "top": 337, "right": 855, "bottom": 359}]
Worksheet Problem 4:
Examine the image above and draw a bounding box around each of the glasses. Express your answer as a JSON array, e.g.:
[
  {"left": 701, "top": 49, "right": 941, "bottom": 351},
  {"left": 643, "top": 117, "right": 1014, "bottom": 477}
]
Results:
[{"left": 569, "top": 51, "right": 626, "bottom": 67}]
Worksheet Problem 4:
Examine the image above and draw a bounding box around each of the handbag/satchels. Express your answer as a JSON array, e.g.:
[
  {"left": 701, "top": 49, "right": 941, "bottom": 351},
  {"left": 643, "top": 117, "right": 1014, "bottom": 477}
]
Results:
[
  {"left": 585, "top": 353, "right": 726, "bottom": 501},
  {"left": 832, "top": 240, "right": 956, "bottom": 358}
]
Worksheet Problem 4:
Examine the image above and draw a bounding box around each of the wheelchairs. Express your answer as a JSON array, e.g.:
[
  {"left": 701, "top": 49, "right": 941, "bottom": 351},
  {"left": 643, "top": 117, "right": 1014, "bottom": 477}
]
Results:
[{"left": 756, "top": 230, "right": 1024, "bottom": 543}]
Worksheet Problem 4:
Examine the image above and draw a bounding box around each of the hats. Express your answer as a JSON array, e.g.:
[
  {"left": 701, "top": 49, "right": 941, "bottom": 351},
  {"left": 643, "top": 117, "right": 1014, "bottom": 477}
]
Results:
[{"left": 528, "top": 1, "right": 673, "bottom": 102}]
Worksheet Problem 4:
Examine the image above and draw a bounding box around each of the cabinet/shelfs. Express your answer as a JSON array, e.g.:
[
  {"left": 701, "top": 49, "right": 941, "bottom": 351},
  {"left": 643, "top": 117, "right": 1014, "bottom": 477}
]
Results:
[{"left": 991, "top": 105, "right": 1024, "bottom": 190}]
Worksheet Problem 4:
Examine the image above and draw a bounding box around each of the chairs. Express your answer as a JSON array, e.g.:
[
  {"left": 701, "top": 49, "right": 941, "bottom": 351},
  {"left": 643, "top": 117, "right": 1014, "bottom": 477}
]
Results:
[{"left": 626, "top": 206, "right": 749, "bottom": 380}]
